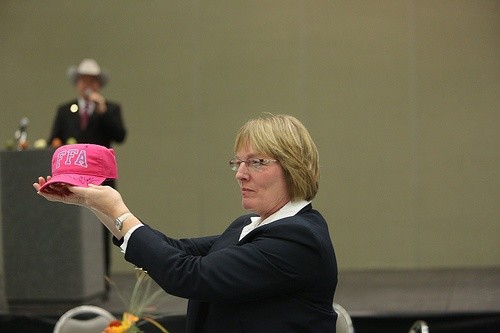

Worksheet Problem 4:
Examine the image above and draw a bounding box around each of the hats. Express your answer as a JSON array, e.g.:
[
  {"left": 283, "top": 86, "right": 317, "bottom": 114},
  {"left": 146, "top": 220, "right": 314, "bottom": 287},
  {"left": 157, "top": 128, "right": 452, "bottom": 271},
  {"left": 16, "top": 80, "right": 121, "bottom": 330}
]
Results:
[
  {"left": 38, "top": 144, "right": 117, "bottom": 192},
  {"left": 69, "top": 58, "right": 108, "bottom": 89}
]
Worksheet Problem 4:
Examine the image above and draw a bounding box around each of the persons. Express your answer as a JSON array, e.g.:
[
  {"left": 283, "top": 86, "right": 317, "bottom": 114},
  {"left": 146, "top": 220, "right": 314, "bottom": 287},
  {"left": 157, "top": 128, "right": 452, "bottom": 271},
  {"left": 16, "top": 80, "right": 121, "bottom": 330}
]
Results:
[
  {"left": 32, "top": 112, "right": 338, "bottom": 333},
  {"left": 46, "top": 58, "right": 126, "bottom": 301}
]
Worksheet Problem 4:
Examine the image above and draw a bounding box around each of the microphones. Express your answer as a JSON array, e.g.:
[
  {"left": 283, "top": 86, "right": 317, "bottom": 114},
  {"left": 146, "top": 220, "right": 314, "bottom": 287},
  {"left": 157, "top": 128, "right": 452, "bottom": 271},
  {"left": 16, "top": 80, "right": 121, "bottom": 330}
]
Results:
[
  {"left": 85, "top": 86, "right": 99, "bottom": 104},
  {"left": 14, "top": 117, "right": 29, "bottom": 140}
]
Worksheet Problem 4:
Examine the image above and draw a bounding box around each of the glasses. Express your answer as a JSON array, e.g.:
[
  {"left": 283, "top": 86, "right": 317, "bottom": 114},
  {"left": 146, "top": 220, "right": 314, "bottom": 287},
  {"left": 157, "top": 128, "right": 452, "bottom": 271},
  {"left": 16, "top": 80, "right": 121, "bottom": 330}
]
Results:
[
  {"left": 229, "top": 157, "right": 277, "bottom": 171},
  {"left": 80, "top": 103, "right": 90, "bottom": 131}
]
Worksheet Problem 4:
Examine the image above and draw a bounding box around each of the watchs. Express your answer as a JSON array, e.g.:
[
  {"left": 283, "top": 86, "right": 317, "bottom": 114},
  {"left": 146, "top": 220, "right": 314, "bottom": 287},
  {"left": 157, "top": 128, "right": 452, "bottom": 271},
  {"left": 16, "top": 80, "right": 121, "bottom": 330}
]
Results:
[{"left": 114, "top": 212, "right": 133, "bottom": 232}]
